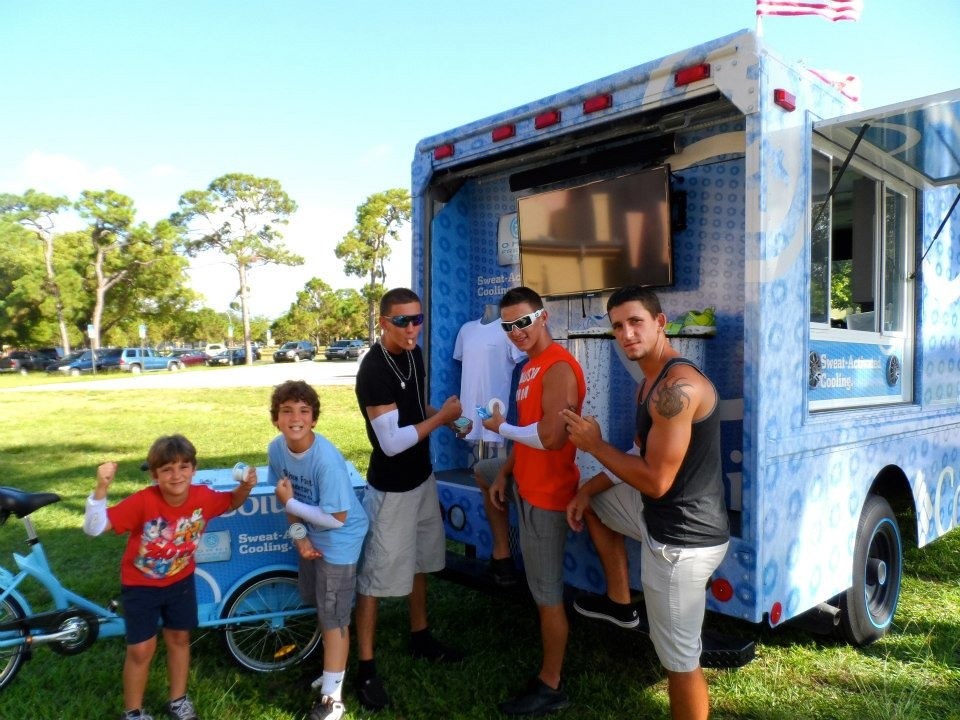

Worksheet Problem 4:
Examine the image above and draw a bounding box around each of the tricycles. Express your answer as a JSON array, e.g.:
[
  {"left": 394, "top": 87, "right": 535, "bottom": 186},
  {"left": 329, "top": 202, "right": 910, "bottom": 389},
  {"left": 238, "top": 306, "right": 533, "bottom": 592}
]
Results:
[{"left": 0, "top": 459, "right": 370, "bottom": 690}]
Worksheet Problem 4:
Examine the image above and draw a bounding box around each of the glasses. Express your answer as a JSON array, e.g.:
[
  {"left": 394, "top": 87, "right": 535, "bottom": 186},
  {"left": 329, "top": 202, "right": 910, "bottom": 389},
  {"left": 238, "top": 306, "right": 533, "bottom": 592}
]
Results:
[
  {"left": 501, "top": 308, "right": 545, "bottom": 333},
  {"left": 381, "top": 314, "right": 424, "bottom": 328}
]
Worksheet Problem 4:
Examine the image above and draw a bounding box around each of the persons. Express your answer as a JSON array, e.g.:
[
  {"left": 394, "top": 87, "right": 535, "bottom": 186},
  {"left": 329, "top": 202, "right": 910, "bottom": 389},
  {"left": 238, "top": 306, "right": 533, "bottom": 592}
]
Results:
[
  {"left": 267, "top": 380, "right": 369, "bottom": 720},
  {"left": 356, "top": 288, "right": 474, "bottom": 710},
  {"left": 474, "top": 287, "right": 586, "bottom": 717},
  {"left": 559, "top": 286, "right": 730, "bottom": 720},
  {"left": 84, "top": 434, "right": 257, "bottom": 720},
  {"left": 453, "top": 304, "right": 527, "bottom": 448}
]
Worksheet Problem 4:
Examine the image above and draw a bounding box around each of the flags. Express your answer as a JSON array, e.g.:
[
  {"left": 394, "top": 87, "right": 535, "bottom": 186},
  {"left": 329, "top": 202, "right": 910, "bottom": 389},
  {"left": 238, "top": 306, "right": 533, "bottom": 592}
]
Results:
[{"left": 755, "top": 0, "right": 860, "bottom": 22}]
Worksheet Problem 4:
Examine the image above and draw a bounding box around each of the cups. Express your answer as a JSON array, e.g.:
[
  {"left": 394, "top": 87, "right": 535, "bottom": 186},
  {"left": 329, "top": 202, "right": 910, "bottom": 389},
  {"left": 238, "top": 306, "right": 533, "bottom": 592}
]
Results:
[
  {"left": 485, "top": 398, "right": 506, "bottom": 415},
  {"left": 454, "top": 418, "right": 470, "bottom": 433},
  {"left": 232, "top": 462, "right": 252, "bottom": 482}
]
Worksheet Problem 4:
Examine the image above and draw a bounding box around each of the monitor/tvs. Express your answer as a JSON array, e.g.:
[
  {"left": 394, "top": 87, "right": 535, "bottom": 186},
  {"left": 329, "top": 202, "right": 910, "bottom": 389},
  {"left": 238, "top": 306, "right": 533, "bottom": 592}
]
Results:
[{"left": 518, "top": 163, "right": 674, "bottom": 300}]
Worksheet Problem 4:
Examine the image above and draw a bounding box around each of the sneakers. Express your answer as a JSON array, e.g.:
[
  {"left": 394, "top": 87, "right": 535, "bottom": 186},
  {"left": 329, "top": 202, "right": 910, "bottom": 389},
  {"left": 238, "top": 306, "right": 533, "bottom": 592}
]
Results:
[
  {"left": 573, "top": 595, "right": 640, "bottom": 629},
  {"left": 122, "top": 709, "right": 153, "bottom": 720},
  {"left": 305, "top": 694, "right": 344, "bottom": 720},
  {"left": 166, "top": 698, "right": 198, "bottom": 720}
]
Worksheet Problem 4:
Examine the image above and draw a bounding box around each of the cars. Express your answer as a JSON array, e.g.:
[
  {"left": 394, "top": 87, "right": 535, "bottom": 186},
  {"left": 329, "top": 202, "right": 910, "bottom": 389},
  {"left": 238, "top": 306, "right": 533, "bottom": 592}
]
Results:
[
  {"left": 324, "top": 339, "right": 366, "bottom": 361},
  {"left": 101, "top": 347, "right": 181, "bottom": 375},
  {"left": 208, "top": 348, "right": 257, "bottom": 367},
  {"left": 273, "top": 341, "right": 316, "bottom": 362},
  {"left": 243, "top": 346, "right": 262, "bottom": 360},
  {"left": 205, "top": 344, "right": 228, "bottom": 357},
  {"left": 45, "top": 348, "right": 112, "bottom": 376},
  {"left": 169, "top": 349, "right": 213, "bottom": 367},
  {"left": 39, "top": 347, "right": 64, "bottom": 360}
]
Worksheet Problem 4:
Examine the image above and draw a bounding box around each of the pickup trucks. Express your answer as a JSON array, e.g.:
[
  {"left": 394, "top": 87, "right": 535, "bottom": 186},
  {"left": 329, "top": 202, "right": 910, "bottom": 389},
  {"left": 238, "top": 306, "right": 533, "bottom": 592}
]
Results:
[{"left": 0, "top": 351, "right": 57, "bottom": 376}]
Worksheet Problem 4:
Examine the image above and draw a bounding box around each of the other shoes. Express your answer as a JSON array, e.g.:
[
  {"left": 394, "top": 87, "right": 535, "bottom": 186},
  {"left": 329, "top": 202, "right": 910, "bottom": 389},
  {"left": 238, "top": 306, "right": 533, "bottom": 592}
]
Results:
[
  {"left": 354, "top": 674, "right": 388, "bottom": 712},
  {"left": 466, "top": 559, "right": 516, "bottom": 585},
  {"left": 407, "top": 636, "right": 467, "bottom": 664},
  {"left": 497, "top": 679, "right": 567, "bottom": 717}
]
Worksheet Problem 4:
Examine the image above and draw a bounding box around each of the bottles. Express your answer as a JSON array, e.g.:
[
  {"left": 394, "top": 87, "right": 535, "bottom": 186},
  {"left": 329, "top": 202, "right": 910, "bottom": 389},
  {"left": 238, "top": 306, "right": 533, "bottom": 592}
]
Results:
[{"left": 475, "top": 404, "right": 492, "bottom": 420}]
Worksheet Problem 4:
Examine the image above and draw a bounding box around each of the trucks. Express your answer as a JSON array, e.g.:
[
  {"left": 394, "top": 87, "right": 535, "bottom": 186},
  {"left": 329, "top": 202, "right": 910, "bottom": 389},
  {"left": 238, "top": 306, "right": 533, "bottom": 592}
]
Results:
[{"left": 408, "top": 28, "right": 960, "bottom": 646}]
